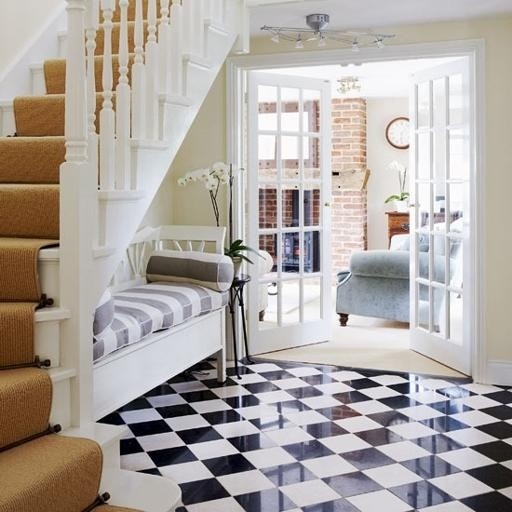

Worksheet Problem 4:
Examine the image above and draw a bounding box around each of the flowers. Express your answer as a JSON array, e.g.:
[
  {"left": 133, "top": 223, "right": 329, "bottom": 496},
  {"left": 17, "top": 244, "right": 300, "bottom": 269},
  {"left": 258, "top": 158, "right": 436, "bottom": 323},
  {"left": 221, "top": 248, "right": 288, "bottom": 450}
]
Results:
[
  {"left": 380, "top": 159, "right": 410, "bottom": 205},
  {"left": 175, "top": 158, "right": 266, "bottom": 268}
]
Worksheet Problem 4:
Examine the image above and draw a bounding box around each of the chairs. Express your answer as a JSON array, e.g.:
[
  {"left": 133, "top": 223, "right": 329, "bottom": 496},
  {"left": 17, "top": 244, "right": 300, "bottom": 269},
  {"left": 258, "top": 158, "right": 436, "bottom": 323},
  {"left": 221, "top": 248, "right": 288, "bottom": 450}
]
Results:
[{"left": 255, "top": 245, "right": 274, "bottom": 323}]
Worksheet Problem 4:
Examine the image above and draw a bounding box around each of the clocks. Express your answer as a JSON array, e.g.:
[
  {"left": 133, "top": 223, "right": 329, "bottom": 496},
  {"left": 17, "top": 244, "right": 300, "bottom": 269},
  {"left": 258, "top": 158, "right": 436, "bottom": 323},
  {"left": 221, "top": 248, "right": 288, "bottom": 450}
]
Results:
[{"left": 385, "top": 116, "right": 411, "bottom": 151}]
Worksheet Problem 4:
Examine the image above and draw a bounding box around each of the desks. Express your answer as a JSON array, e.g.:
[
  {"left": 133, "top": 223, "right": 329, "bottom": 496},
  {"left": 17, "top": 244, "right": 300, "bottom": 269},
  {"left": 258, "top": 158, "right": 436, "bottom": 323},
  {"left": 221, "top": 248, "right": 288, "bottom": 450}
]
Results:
[{"left": 225, "top": 272, "right": 256, "bottom": 381}]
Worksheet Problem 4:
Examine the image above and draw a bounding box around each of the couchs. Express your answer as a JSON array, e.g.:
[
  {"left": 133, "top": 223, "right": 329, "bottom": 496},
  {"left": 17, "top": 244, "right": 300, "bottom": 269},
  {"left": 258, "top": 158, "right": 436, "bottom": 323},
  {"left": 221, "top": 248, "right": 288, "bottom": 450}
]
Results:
[{"left": 336, "top": 215, "right": 467, "bottom": 334}]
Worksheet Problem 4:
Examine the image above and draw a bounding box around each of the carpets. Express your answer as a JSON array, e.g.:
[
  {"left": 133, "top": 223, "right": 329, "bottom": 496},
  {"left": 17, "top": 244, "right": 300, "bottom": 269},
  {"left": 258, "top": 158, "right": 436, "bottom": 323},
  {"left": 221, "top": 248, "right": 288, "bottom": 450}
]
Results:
[{"left": 260, "top": 280, "right": 322, "bottom": 316}]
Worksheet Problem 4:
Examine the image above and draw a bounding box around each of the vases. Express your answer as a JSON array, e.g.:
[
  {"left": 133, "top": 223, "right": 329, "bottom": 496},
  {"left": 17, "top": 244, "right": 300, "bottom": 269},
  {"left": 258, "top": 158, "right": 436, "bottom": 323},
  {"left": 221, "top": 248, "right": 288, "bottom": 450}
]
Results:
[
  {"left": 395, "top": 200, "right": 410, "bottom": 214},
  {"left": 232, "top": 255, "right": 242, "bottom": 277}
]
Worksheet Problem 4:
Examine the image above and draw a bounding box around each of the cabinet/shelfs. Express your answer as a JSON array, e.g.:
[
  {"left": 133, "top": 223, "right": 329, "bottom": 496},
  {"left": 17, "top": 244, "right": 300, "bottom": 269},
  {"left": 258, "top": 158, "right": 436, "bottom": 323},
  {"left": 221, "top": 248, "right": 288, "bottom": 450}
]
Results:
[
  {"left": 383, "top": 209, "right": 463, "bottom": 250},
  {"left": 272, "top": 228, "right": 321, "bottom": 276}
]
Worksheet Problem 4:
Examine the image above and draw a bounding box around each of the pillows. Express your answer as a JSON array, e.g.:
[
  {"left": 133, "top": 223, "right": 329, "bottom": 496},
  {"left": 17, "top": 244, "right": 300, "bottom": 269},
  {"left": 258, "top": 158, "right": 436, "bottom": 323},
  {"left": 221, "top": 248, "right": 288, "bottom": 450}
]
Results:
[
  {"left": 94, "top": 286, "right": 117, "bottom": 346},
  {"left": 142, "top": 247, "right": 236, "bottom": 294}
]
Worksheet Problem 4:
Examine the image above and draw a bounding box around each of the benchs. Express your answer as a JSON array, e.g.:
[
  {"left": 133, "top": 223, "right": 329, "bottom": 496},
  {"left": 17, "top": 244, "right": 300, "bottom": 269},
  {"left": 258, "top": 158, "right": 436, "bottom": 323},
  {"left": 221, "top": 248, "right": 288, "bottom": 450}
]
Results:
[{"left": 85, "top": 222, "right": 234, "bottom": 425}]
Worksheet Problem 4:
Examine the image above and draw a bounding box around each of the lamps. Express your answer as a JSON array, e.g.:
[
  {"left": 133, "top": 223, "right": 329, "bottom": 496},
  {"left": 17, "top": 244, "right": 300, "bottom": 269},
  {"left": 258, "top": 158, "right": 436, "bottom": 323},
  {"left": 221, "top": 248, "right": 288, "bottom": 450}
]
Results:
[
  {"left": 258, "top": 11, "right": 399, "bottom": 54},
  {"left": 324, "top": 74, "right": 366, "bottom": 96}
]
